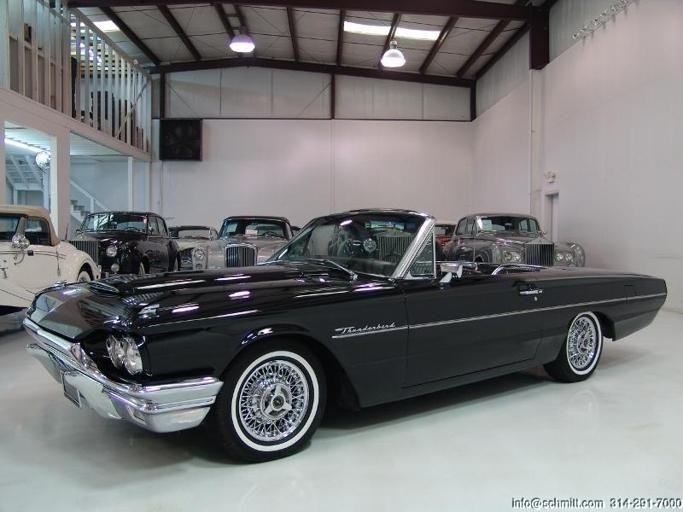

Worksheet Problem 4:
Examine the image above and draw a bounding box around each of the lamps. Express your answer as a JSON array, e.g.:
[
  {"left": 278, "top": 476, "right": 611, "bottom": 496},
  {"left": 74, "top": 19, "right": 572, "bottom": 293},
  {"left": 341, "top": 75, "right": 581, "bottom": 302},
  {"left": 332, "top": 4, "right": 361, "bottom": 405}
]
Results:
[
  {"left": 380, "top": 39, "right": 406, "bottom": 68},
  {"left": 228, "top": 25, "right": 256, "bottom": 53}
]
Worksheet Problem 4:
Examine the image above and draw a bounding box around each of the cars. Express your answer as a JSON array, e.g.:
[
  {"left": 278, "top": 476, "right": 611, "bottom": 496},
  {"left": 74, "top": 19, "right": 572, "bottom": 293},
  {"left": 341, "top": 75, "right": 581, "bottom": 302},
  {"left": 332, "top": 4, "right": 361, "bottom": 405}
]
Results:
[
  {"left": 436, "top": 212, "right": 586, "bottom": 268},
  {"left": 0, "top": 203, "right": 298, "bottom": 318},
  {"left": 21, "top": 206, "right": 666, "bottom": 464}
]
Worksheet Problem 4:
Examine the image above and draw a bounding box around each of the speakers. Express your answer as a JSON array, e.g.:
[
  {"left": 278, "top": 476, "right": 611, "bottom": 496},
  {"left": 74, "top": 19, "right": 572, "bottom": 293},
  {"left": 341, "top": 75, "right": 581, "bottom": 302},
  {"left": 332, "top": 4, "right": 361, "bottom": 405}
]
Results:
[{"left": 159, "top": 118, "right": 202, "bottom": 161}]
